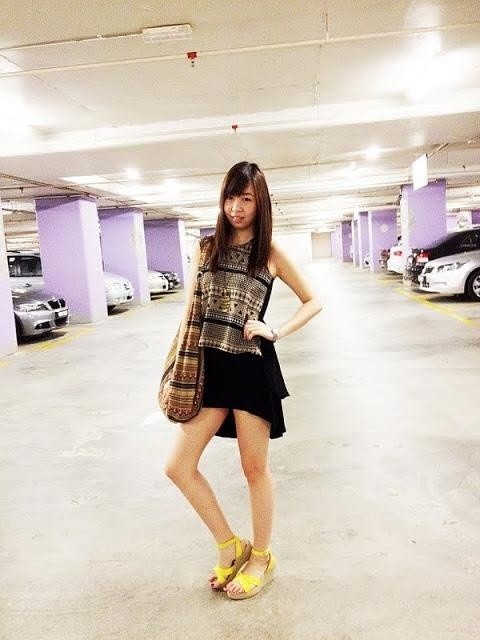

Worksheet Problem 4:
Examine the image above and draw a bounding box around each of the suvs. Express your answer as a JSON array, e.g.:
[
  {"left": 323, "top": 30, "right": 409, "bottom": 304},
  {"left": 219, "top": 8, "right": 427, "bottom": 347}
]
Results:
[{"left": 7, "top": 248, "right": 135, "bottom": 313}]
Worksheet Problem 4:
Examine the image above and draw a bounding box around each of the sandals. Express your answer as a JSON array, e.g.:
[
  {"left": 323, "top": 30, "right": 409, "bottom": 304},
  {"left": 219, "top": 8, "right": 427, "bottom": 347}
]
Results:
[
  {"left": 226, "top": 546, "right": 275, "bottom": 600},
  {"left": 207, "top": 535, "right": 252, "bottom": 590}
]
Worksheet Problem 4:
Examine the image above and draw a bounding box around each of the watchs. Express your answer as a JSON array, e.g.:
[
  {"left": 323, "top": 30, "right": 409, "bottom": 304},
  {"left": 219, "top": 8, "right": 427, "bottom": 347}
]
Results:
[{"left": 271, "top": 327, "right": 279, "bottom": 343}]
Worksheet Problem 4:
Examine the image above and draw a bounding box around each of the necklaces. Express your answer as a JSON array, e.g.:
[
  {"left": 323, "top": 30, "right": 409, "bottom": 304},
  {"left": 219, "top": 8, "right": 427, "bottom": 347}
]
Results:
[{"left": 221, "top": 240, "right": 253, "bottom": 290}]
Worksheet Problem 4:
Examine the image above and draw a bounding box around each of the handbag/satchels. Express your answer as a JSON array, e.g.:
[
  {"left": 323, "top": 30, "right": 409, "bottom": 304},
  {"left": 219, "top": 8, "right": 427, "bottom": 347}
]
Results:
[{"left": 158, "top": 291, "right": 207, "bottom": 425}]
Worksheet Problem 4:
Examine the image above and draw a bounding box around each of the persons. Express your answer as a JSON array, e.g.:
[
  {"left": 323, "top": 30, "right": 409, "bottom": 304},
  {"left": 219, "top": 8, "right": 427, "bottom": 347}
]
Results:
[{"left": 157, "top": 161, "right": 322, "bottom": 604}]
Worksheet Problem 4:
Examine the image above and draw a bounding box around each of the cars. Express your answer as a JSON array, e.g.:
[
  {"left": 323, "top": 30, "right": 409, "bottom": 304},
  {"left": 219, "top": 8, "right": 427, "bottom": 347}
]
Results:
[
  {"left": 11, "top": 283, "right": 71, "bottom": 338},
  {"left": 148, "top": 269, "right": 179, "bottom": 294},
  {"left": 349, "top": 227, "right": 480, "bottom": 301}
]
